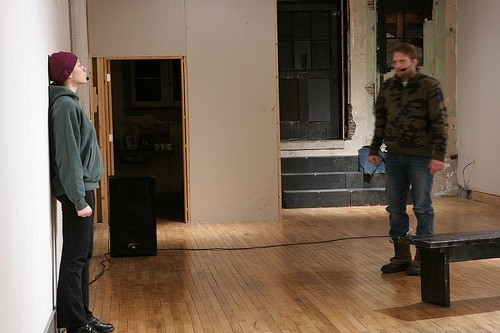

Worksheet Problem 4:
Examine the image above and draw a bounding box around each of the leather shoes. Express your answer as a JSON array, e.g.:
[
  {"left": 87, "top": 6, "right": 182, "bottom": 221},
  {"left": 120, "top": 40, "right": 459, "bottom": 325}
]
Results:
[
  {"left": 65, "top": 324, "right": 100, "bottom": 333},
  {"left": 89, "top": 318, "right": 116, "bottom": 333}
]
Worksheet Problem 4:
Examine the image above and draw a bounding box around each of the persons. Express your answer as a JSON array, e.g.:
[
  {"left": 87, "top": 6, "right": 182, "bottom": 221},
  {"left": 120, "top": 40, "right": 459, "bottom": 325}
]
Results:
[
  {"left": 367, "top": 42, "right": 449, "bottom": 277},
  {"left": 48, "top": 50, "right": 115, "bottom": 333}
]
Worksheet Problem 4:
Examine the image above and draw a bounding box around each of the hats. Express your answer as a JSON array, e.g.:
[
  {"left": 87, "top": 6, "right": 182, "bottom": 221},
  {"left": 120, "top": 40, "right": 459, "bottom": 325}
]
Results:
[{"left": 48, "top": 51, "right": 79, "bottom": 86}]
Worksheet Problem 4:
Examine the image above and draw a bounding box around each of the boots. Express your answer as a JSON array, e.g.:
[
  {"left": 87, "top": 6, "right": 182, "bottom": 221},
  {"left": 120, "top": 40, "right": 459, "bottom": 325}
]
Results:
[
  {"left": 407, "top": 246, "right": 420, "bottom": 275},
  {"left": 381, "top": 227, "right": 412, "bottom": 272}
]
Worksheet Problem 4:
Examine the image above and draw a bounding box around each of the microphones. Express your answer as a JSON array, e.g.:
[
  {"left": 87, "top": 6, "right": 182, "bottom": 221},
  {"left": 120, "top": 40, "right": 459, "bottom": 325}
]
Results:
[
  {"left": 86, "top": 77, "right": 89, "bottom": 80},
  {"left": 400, "top": 65, "right": 412, "bottom": 72}
]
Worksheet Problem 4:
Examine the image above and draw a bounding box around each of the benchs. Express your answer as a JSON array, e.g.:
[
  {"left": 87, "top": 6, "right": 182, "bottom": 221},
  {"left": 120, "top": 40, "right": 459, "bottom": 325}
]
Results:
[{"left": 397, "top": 229, "right": 500, "bottom": 308}]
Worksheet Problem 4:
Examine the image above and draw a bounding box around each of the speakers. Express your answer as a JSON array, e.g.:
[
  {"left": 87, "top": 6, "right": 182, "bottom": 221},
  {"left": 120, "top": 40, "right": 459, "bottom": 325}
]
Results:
[{"left": 109, "top": 176, "right": 158, "bottom": 258}]
